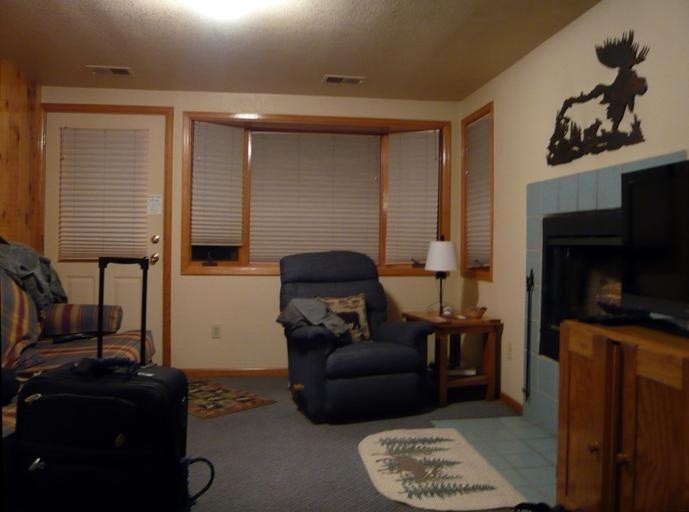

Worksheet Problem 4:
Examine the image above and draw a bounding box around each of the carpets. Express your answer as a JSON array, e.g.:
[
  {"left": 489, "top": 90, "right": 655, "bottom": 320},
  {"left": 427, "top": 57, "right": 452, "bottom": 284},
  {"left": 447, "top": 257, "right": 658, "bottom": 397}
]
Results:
[
  {"left": 356, "top": 429, "right": 526, "bottom": 510},
  {"left": 186, "top": 379, "right": 278, "bottom": 420}
]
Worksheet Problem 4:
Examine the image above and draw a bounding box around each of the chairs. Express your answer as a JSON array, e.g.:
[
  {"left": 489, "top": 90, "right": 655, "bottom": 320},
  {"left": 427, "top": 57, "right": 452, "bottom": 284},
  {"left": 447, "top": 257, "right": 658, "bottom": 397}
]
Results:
[{"left": 279, "top": 250, "right": 435, "bottom": 426}]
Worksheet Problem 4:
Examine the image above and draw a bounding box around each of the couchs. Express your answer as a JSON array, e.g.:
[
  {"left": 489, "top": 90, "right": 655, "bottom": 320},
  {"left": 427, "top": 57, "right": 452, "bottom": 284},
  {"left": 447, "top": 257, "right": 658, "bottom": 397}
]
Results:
[{"left": 0, "top": 247, "right": 157, "bottom": 442}]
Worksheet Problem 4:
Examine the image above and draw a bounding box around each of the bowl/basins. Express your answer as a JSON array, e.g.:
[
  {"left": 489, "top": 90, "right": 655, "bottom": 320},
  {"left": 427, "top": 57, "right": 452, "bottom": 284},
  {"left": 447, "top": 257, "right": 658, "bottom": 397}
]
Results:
[{"left": 462, "top": 304, "right": 486, "bottom": 319}]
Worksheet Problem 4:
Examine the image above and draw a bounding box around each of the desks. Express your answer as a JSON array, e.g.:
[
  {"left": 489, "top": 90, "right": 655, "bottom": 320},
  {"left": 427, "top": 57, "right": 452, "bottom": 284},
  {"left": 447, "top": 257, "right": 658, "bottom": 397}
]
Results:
[{"left": 402, "top": 307, "right": 503, "bottom": 408}]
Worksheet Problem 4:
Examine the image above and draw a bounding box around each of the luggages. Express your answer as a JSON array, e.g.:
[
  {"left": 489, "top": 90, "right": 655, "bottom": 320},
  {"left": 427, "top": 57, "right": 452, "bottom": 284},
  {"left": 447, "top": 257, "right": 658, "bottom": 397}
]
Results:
[{"left": 15, "top": 253, "right": 188, "bottom": 512}]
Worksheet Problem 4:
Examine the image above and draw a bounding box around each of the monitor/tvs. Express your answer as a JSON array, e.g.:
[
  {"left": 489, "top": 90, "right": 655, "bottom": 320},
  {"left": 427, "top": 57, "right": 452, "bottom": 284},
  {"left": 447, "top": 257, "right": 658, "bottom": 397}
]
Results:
[{"left": 617, "top": 159, "right": 689, "bottom": 335}]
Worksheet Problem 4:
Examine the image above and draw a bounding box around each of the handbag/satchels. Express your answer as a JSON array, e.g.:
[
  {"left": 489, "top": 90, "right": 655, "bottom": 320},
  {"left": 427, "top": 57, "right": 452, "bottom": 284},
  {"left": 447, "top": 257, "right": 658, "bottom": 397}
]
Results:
[{"left": 276, "top": 298, "right": 348, "bottom": 337}]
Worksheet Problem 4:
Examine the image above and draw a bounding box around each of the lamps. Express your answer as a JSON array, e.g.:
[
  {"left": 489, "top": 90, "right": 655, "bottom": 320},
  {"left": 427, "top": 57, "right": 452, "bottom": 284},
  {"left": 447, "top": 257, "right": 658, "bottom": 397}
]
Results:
[{"left": 424, "top": 234, "right": 460, "bottom": 318}]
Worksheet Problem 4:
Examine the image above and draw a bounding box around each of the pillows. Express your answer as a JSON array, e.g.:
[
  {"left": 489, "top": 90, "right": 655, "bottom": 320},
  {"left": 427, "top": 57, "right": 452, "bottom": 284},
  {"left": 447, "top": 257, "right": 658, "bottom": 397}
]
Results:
[
  {"left": 314, "top": 294, "right": 373, "bottom": 345},
  {"left": 0, "top": 267, "right": 44, "bottom": 369}
]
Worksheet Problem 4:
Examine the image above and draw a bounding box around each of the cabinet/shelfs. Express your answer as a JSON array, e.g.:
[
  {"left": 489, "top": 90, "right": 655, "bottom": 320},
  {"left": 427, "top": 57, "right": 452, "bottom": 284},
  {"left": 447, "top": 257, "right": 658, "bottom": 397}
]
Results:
[{"left": 553, "top": 319, "right": 689, "bottom": 511}]
